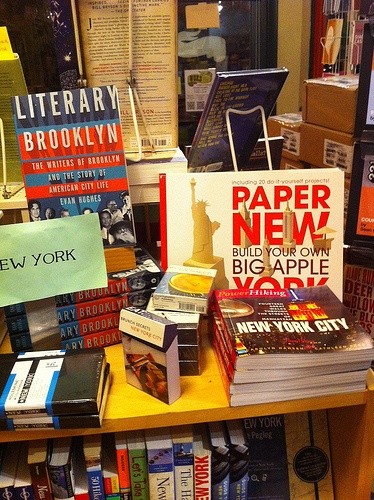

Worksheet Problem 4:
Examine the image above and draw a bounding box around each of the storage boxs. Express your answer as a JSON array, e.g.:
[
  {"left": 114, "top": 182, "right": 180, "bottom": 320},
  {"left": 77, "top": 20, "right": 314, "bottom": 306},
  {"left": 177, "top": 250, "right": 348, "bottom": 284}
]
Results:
[
  {"left": 119, "top": 264, "right": 218, "bottom": 406},
  {"left": 265, "top": 73, "right": 361, "bottom": 236}
]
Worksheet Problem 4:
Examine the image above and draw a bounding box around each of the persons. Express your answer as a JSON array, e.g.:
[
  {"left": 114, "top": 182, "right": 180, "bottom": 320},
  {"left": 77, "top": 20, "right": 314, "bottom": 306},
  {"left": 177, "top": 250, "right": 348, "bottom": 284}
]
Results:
[
  {"left": 189, "top": 177, "right": 221, "bottom": 264},
  {"left": 29, "top": 190, "right": 134, "bottom": 245}
]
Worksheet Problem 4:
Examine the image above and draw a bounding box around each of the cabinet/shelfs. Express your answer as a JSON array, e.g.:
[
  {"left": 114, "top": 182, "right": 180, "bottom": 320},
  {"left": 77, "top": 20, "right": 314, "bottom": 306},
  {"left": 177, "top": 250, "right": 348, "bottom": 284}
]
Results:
[{"left": 1, "top": 318, "right": 374, "bottom": 500}]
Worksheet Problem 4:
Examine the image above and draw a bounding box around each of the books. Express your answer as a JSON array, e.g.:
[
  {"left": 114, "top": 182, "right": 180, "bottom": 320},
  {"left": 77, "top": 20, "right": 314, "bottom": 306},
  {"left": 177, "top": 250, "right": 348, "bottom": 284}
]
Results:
[
  {"left": 0, "top": 247, "right": 374, "bottom": 425},
  {"left": 160, "top": 169, "right": 344, "bottom": 304},
  {"left": 0, "top": 424, "right": 248, "bottom": 500},
  {"left": 188, "top": 66, "right": 290, "bottom": 173},
  {"left": 12, "top": 84, "right": 138, "bottom": 248}
]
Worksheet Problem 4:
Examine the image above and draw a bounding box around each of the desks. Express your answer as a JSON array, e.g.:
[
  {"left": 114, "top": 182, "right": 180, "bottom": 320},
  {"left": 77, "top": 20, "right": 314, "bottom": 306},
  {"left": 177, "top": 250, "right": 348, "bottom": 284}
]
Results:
[{"left": 0, "top": 184, "right": 30, "bottom": 223}]
